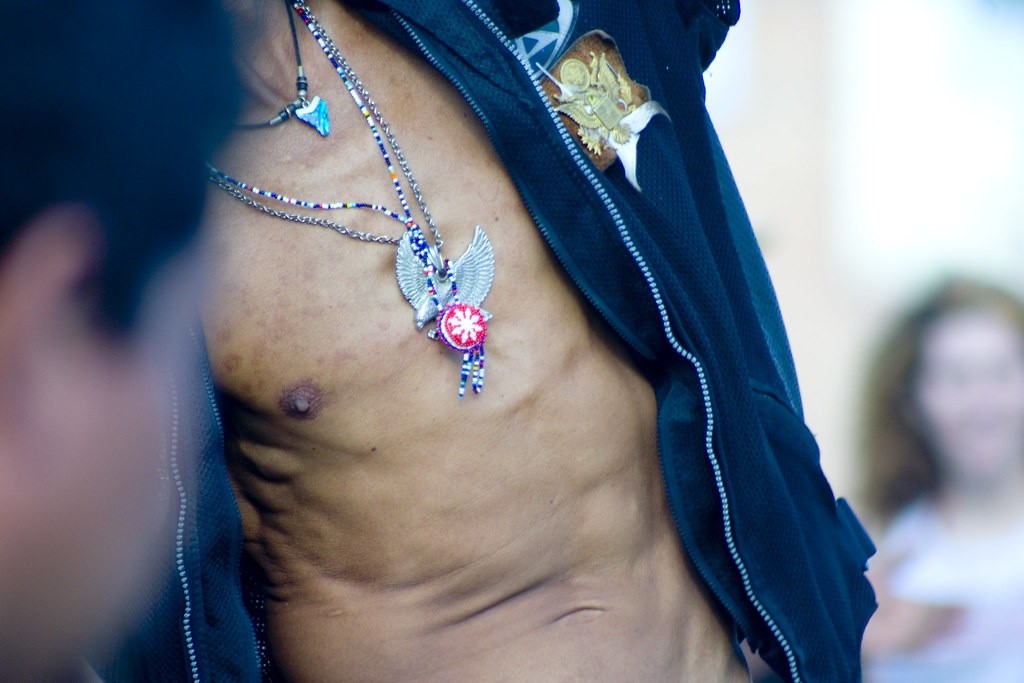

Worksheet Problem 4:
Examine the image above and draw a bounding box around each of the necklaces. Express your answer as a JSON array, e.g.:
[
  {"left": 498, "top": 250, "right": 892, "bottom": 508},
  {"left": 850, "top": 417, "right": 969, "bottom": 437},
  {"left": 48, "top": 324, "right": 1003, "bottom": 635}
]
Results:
[{"left": 206, "top": 0, "right": 495, "bottom": 398}]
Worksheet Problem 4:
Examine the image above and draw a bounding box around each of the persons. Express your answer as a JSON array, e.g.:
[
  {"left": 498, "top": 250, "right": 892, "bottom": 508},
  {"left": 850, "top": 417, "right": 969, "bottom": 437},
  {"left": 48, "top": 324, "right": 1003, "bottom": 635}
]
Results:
[
  {"left": 865, "top": 281, "right": 1024, "bottom": 683},
  {"left": 0, "top": 0, "right": 878, "bottom": 683}
]
